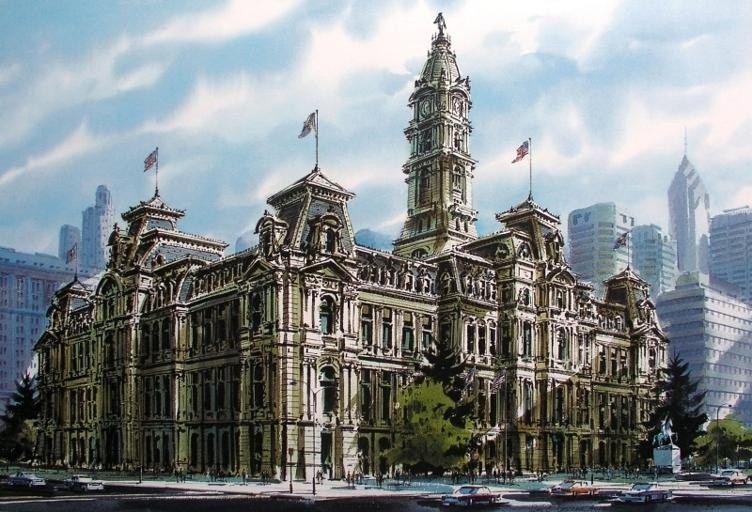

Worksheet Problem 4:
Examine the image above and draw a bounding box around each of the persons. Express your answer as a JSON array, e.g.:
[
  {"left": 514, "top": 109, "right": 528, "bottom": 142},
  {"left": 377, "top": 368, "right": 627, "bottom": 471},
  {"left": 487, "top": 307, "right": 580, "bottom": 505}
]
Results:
[
  {"left": 450, "top": 470, "right": 477, "bottom": 486},
  {"left": 376, "top": 472, "right": 383, "bottom": 488},
  {"left": 316, "top": 469, "right": 323, "bottom": 482},
  {"left": 262, "top": 470, "right": 270, "bottom": 484},
  {"left": 395, "top": 468, "right": 409, "bottom": 482},
  {"left": 242, "top": 469, "right": 246, "bottom": 483},
  {"left": 660, "top": 419, "right": 668, "bottom": 440},
  {"left": 174, "top": 467, "right": 186, "bottom": 484},
  {"left": 566, "top": 466, "right": 587, "bottom": 480},
  {"left": 597, "top": 465, "right": 640, "bottom": 480},
  {"left": 494, "top": 469, "right": 512, "bottom": 484},
  {"left": 347, "top": 470, "right": 364, "bottom": 487},
  {"left": 209, "top": 466, "right": 223, "bottom": 482}
]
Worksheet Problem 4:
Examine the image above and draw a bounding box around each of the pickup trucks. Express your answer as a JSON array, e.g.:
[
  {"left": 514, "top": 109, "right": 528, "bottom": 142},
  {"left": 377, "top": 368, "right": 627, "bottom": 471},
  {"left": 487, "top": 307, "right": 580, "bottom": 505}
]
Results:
[
  {"left": 7, "top": 472, "right": 45, "bottom": 488},
  {"left": 610, "top": 483, "right": 672, "bottom": 506},
  {"left": 64, "top": 475, "right": 103, "bottom": 493}
]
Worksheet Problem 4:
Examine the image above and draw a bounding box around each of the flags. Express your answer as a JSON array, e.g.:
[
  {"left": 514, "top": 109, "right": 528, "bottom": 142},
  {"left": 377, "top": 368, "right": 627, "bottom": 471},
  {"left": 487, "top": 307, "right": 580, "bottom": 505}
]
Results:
[
  {"left": 511, "top": 140, "right": 529, "bottom": 163},
  {"left": 463, "top": 366, "right": 474, "bottom": 390},
  {"left": 492, "top": 370, "right": 505, "bottom": 393},
  {"left": 612, "top": 231, "right": 627, "bottom": 251},
  {"left": 144, "top": 149, "right": 157, "bottom": 170},
  {"left": 65, "top": 244, "right": 76, "bottom": 264},
  {"left": 297, "top": 112, "right": 317, "bottom": 138}
]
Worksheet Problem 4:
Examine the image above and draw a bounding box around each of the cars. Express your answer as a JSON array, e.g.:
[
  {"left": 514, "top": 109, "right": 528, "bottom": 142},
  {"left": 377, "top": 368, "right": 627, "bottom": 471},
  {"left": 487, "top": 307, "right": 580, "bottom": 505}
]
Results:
[
  {"left": 675, "top": 471, "right": 716, "bottom": 481},
  {"left": 440, "top": 485, "right": 502, "bottom": 507},
  {"left": 712, "top": 470, "right": 751, "bottom": 487},
  {"left": 550, "top": 480, "right": 601, "bottom": 498}
]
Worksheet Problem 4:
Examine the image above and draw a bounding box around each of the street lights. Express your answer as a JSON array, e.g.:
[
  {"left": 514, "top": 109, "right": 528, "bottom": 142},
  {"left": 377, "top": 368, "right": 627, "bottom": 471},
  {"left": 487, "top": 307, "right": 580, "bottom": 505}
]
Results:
[
  {"left": 716, "top": 403, "right": 733, "bottom": 473},
  {"left": 289, "top": 379, "right": 341, "bottom": 494},
  {"left": 121, "top": 400, "right": 142, "bottom": 482}
]
